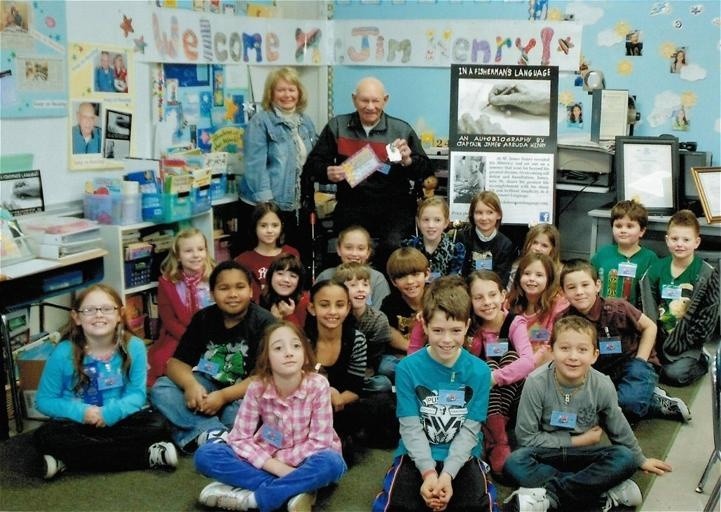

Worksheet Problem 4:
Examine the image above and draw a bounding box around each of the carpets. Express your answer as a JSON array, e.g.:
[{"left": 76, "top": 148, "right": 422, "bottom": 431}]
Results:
[{"left": 2, "top": 354, "right": 710, "bottom": 512}]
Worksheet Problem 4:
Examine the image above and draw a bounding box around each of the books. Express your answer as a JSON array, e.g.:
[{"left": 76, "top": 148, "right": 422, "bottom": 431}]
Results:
[
  {"left": 20, "top": 214, "right": 101, "bottom": 241},
  {"left": 29, "top": 242, "right": 99, "bottom": 260}
]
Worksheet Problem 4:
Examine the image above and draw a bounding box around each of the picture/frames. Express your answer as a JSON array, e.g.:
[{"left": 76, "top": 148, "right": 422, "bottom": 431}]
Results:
[
  {"left": 614, "top": 133, "right": 682, "bottom": 218},
  {"left": 690, "top": 164, "right": 721, "bottom": 225}
]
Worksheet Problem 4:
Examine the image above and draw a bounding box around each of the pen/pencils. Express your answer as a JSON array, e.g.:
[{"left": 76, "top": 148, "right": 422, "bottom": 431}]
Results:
[{"left": 479, "top": 83, "right": 517, "bottom": 111}]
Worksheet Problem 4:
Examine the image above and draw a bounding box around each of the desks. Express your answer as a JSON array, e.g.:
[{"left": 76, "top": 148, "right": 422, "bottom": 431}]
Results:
[{"left": 585, "top": 198, "right": 721, "bottom": 277}]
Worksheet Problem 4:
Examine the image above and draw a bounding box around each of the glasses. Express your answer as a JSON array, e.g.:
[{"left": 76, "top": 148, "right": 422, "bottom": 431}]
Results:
[{"left": 78, "top": 304, "right": 119, "bottom": 315}]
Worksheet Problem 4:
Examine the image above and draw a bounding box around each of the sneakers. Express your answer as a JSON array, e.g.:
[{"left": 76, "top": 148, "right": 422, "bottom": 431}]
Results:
[
  {"left": 33, "top": 454, "right": 64, "bottom": 479},
  {"left": 651, "top": 387, "right": 691, "bottom": 422},
  {"left": 199, "top": 482, "right": 251, "bottom": 511},
  {"left": 198, "top": 428, "right": 229, "bottom": 444},
  {"left": 147, "top": 442, "right": 178, "bottom": 468},
  {"left": 601, "top": 478, "right": 643, "bottom": 512},
  {"left": 517, "top": 485, "right": 550, "bottom": 512},
  {"left": 288, "top": 488, "right": 317, "bottom": 512}
]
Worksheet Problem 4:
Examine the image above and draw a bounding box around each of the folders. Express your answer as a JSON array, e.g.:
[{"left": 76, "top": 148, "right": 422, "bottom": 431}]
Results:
[
  {"left": 21, "top": 217, "right": 102, "bottom": 245},
  {"left": 25, "top": 239, "right": 102, "bottom": 260}
]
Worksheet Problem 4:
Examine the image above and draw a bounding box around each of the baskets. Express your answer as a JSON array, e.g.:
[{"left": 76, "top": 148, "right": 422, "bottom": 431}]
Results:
[{"left": 125, "top": 256, "right": 152, "bottom": 287}]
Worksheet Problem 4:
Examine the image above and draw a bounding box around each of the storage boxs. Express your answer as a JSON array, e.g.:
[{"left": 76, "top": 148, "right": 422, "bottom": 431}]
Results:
[{"left": 78, "top": 163, "right": 231, "bottom": 227}]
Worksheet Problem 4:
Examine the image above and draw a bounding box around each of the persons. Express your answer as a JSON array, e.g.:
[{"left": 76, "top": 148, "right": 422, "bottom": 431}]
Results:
[
  {"left": 301, "top": 76, "right": 431, "bottom": 249},
  {"left": 487, "top": 81, "right": 549, "bottom": 117},
  {"left": 574, "top": 64, "right": 590, "bottom": 86},
  {"left": 73, "top": 103, "right": 101, "bottom": 154},
  {"left": 93, "top": 52, "right": 115, "bottom": 92},
  {"left": 456, "top": 160, "right": 485, "bottom": 195},
  {"left": 672, "top": 50, "right": 686, "bottom": 73},
  {"left": 570, "top": 104, "right": 583, "bottom": 127},
  {"left": 111, "top": 55, "right": 127, "bottom": 92},
  {"left": 30, "top": 191, "right": 720, "bottom": 510},
  {"left": 675, "top": 109, "right": 689, "bottom": 131},
  {"left": 233, "top": 67, "right": 320, "bottom": 257},
  {"left": 625, "top": 33, "right": 642, "bottom": 56}
]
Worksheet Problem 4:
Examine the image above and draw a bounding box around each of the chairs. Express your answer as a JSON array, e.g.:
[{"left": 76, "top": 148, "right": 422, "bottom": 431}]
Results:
[{"left": 695, "top": 342, "right": 721, "bottom": 512}]
